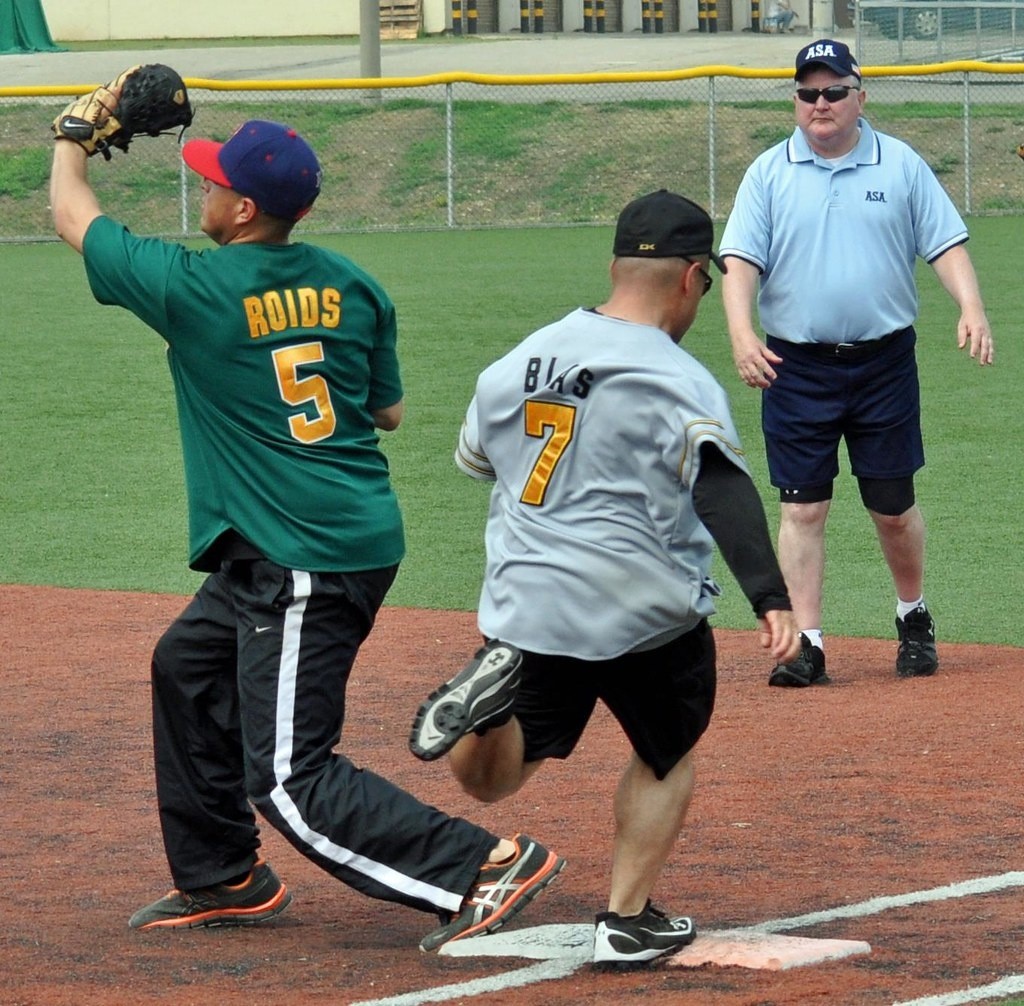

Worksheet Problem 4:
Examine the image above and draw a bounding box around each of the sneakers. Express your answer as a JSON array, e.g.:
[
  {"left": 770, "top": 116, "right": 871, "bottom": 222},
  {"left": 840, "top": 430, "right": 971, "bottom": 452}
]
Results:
[
  {"left": 409, "top": 637, "right": 525, "bottom": 761},
  {"left": 126, "top": 852, "right": 294, "bottom": 933},
  {"left": 418, "top": 831, "right": 569, "bottom": 955},
  {"left": 591, "top": 897, "right": 696, "bottom": 970},
  {"left": 768, "top": 631, "right": 830, "bottom": 688},
  {"left": 893, "top": 605, "right": 939, "bottom": 677}
]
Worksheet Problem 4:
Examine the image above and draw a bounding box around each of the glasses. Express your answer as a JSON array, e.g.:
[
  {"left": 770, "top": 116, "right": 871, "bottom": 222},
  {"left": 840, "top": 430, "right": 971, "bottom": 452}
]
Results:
[
  {"left": 679, "top": 254, "right": 713, "bottom": 296},
  {"left": 796, "top": 84, "right": 861, "bottom": 103}
]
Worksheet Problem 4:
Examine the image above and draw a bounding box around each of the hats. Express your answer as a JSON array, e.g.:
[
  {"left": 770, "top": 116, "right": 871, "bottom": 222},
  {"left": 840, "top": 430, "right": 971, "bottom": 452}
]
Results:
[
  {"left": 182, "top": 120, "right": 323, "bottom": 220},
  {"left": 611, "top": 188, "right": 729, "bottom": 277},
  {"left": 793, "top": 39, "right": 863, "bottom": 85}
]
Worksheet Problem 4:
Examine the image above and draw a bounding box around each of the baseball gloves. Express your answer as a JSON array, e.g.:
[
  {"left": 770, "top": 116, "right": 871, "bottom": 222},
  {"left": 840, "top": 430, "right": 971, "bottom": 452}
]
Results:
[{"left": 51, "top": 62, "right": 195, "bottom": 162}]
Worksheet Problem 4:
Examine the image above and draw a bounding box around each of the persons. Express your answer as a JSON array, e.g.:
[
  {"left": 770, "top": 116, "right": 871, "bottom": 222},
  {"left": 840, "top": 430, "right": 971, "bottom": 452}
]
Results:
[
  {"left": 46, "top": 62, "right": 566, "bottom": 953},
  {"left": 406, "top": 188, "right": 801, "bottom": 964},
  {"left": 718, "top": 41, "right": 993, "bottom": 685}
]
